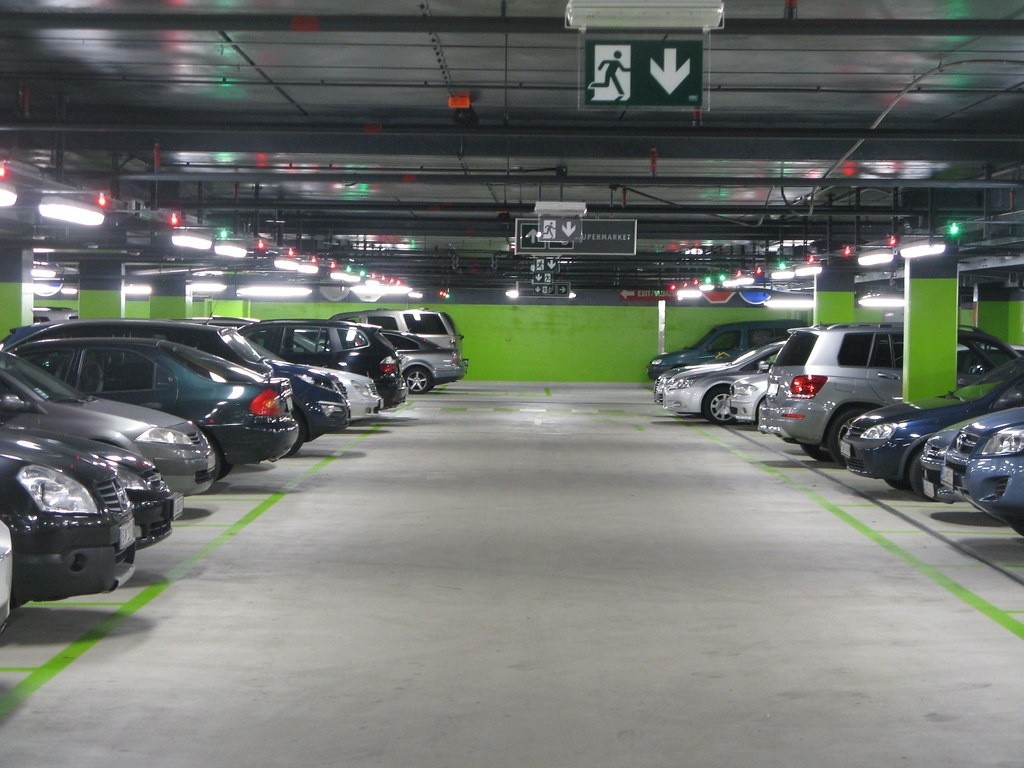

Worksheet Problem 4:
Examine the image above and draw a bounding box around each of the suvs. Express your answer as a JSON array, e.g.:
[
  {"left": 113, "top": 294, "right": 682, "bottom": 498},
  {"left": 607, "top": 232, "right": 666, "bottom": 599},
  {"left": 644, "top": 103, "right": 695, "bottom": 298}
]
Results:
[
  {"left": 646, "top": 319, "right": 807, "bottom": 380},
  {"left": 329, "top": 307, "right": 469, "bottom": 379},
  {"left": 756, "top": 321, "right": 1023, "bottom": 471},
  {"left": 236, "top": 318, "right": 409, "bottom": 411},
  {"left": 169, "top": 316, "right": 328, "bottom": 355}
]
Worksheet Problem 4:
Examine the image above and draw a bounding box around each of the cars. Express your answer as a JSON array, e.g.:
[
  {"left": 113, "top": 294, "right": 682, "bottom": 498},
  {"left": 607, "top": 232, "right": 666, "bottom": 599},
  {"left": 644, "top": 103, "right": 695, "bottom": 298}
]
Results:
[
  {"left": 378, "top": 327, "right": 463, "bottom": 396},
  {"left": 653, "top": 339, "right": 788, "bottom": 425},
  {"left": 7, "top": 336, "right": 298, "bottom": 482},
  {"left": 921, "top": 406, "right": 1024, "bottom": 540},
  {"left": 297, "top": 366, "right": 385, "bottom": 421},
  {"left": 0, "top": 316, "right": 351, "bottom": 459},
  {"left": 838, "top": 355, "right": 1024, "bottom": 503},
  {"left": 0, "top": 420, "right": 185, "bottom": 553},
  {"left": 0, "top": 427, "right": 136, "bottom": 610},
  {"left": 727, "top": 373, "right": 779, "bottom": 425},
  {"left": 0, "top": 351, "right": 217, "bottom": 498},
  {"left": 32, "top": 306, "right": 80, "bottom": 325}
]
